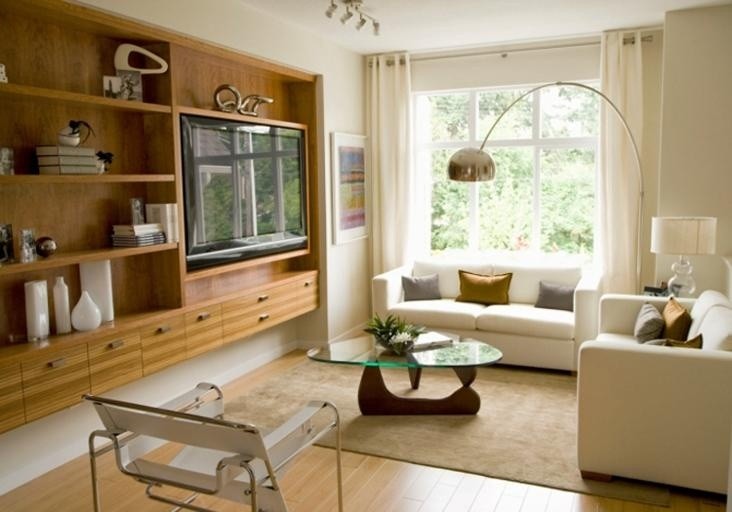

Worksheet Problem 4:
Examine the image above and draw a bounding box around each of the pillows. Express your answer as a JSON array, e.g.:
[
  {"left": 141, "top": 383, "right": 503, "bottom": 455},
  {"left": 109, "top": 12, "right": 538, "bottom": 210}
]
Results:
[
  {"left": 454, "top": 268, "right": 515, "bottom": 305},
  {"left": 662, "top": 332, "right": 703, "bottom": 352},
  {"left": 400, "top": 273, "right": 443, "bottom": 304},
  {"left": 660, "top": 296, "right": 693, "bottom": 342},
  {"left": 633, "top": 301, "right": 664, "bottom": 343},
  {"left": 534, "top": 280, "right": 575, "bottom": 315}
]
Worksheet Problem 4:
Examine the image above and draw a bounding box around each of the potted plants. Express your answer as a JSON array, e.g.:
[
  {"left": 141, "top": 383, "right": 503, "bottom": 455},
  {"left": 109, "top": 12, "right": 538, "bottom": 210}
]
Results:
[{"left": 363, "top": 313, "right": 425, "bottom": 355}]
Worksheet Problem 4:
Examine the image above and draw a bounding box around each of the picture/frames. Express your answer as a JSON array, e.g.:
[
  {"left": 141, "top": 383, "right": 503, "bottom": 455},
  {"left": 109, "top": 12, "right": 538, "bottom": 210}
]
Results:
[{"left": 328, "top": 129, "right": 372, "bottom": 246}]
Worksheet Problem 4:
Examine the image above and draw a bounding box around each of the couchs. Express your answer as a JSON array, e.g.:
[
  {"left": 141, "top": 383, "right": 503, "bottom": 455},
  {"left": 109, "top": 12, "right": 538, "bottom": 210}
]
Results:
[
  {"left": 369, "top": 260, "right": 603, "bottom": 376},
  {"left": 575, "top": 286, "right": 731, "bottom": 498}
]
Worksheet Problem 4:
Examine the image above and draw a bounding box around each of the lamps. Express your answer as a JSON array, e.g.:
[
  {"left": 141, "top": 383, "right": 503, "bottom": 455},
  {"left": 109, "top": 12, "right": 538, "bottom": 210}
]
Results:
[
  {"left": 323, "top": 0, "right": 382, "bottom": 37},
  {"left": 645, "top": 213, "right": 720, "bottom": 297},
  {"left": 443, "top": 80, "right": 645, "bottom": 295}
]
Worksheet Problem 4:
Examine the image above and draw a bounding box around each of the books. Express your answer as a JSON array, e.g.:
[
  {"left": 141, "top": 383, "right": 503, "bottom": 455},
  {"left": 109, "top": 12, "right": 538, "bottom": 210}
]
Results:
[
  {"left": 35, "top": 144, "right": 98, "bottom": 176},
  {"left": 111, "top": 202, "right": 178, "bottom": 247}
]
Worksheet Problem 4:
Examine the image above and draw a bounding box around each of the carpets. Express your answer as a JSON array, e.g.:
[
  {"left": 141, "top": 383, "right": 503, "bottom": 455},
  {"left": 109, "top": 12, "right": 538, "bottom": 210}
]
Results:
[{"left": 220, "top": 358, "right": 677, "bottom": 510}]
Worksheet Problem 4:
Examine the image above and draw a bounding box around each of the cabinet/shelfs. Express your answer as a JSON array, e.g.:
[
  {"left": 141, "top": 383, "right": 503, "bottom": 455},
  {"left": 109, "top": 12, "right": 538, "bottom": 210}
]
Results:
[{"left": 0, "top": 0, "right": 322, "bottom": 434}]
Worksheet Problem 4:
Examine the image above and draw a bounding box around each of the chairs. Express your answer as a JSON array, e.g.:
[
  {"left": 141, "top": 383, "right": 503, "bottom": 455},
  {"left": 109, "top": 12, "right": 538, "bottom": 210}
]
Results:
[{"left": 81, "top": 378, "right": 345, "bottom": 512}]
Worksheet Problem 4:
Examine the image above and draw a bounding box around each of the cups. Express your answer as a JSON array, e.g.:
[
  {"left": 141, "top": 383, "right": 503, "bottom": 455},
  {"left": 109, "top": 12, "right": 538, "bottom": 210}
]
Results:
[
  {"left": 78, "top": 259, "right": 115, "bottom": 322},
  {"left": 0, "top": 147, "right": 15, "bottom": 176},
  {"left": 24, "top": 278, "right": 51, "bottom": 340}
]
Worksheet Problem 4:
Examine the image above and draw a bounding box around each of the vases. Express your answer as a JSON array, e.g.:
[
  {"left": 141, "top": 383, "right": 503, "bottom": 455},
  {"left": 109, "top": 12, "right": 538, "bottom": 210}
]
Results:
[{"left": 378, "top": 340, "right": 414, "bottom": 355}]
